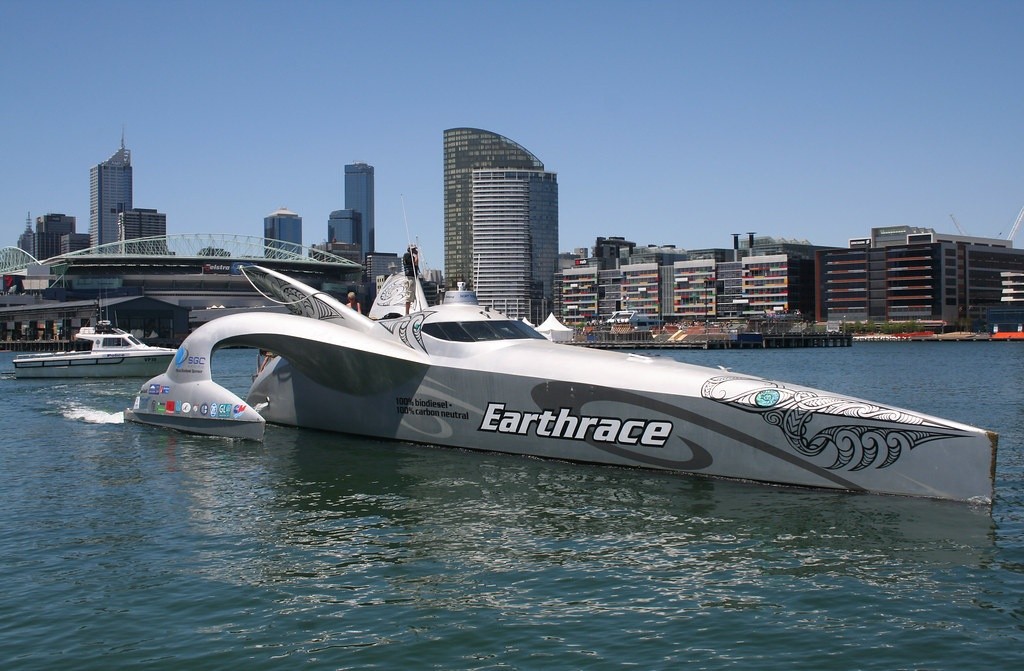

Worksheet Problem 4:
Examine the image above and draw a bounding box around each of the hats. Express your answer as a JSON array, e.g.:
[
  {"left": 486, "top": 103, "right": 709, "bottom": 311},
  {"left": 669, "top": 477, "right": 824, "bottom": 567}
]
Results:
[{"left": 407, "top": 243, "right": 417, "bottom": 250}]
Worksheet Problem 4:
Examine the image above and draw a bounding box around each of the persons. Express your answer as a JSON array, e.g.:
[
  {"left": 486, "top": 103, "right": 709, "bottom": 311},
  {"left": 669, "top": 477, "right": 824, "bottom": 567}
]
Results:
[
  {"left": 4, "top": 275, "right": 13, "bottom": 294},
  {"left": 347, "top": 292, "right": 361, "bottom": 314},
  {"left": 662, "top": 319, "right": 734, "bottom": 332},
  {"left": 403, "top": 244, "right": 420, "bottom": 315}
]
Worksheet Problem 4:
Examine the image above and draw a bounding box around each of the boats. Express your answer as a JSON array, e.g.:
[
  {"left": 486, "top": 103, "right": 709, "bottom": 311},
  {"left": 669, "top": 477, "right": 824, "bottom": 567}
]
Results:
[
  {"left": 11, "top": 316, "right": 179, "bottom": 378},
  {"left": 121, "top": 262, "right": 1002, "bottom": 506}
]
